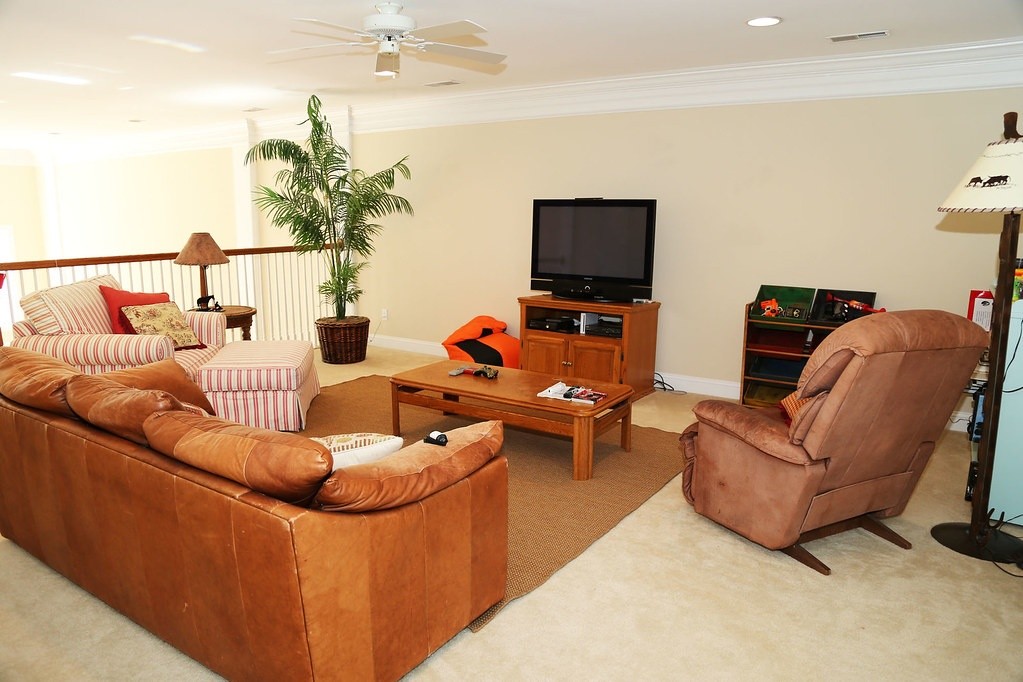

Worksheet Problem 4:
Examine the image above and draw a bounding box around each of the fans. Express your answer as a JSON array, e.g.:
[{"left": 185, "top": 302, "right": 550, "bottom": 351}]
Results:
[{"left": 263, "top": 2, "right": 507, "bottom": 64}]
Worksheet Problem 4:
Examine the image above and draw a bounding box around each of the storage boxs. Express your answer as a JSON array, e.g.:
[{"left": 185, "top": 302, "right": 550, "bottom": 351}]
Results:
[
  {"left": 966, "top": 289, "right": 994, "bottom": 332},
  {"left": 750, "top": 285, "right": 817, "bottom": 325},
  {"left": 807, "top": 288, "right": 877, "bottom": 328}
]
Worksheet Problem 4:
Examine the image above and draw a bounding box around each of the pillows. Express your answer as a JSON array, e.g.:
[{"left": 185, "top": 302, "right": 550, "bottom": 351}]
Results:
[
  {"left": 99, "top": 285, "right": 171, "bottom": 335},
  {"left": 780, "top": 388, "right": 814, "bottom": 420},
  {"left": 309, "top": 433, "right": 404, "bottom": 474},
  {"left": 118, "top": 301, "right": 208, "bottom": 351}
]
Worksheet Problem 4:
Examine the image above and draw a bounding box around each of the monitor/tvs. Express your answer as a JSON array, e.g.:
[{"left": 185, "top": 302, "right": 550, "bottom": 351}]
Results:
[{"left": 531, "top": 199, "right": 657, "bottom": 303}]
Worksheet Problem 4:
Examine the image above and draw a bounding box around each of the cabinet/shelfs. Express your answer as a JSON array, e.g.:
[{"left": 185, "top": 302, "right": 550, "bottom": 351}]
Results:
[
  {"left": 738, "top": 284, "right": 877, "bottom": 410},
  {"left": 517, "top": 293, "right": 661, "bottom": 403}
]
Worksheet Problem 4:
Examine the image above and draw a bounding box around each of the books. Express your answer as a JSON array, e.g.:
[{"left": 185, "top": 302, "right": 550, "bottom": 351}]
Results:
[{"left": 571, "top": 390, "right": 608, "bottom": 404}]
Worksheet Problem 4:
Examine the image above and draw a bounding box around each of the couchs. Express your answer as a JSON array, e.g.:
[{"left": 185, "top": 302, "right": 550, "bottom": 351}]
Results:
[
  {"left": 7, "top": 274, "right": 227, "bottom": 384},
  {"left": 0, "top": 345, "right": 507, "bottom": 682},
  {"left": 679, "top": 310, "right": 989, "bottom": 575},
  {"left": 196, "top": 340, "right": 321, "bottom": 431}
]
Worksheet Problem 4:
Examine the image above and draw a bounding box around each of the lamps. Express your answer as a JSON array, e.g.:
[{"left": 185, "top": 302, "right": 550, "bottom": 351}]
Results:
[
  {"left": 173, "top": 232, "right": 230, "bottom": 310},
  {"left": 928, "top": 110, "right": 1023, "bottom": 566},
  {"left": 374, "top": 41, "right": 401, "bottom": 78}
]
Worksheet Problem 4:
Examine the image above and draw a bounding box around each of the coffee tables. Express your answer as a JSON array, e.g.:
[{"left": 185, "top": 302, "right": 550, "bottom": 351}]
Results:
[{"left": 389, "top": 360, "right": 634, "bottom": 481}]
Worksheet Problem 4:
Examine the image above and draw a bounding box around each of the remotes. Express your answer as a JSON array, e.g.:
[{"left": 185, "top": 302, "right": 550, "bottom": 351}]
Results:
[
  {"left": 449, "top": 365, "right": 468, "bottom": 375},
  {"left": 563, "top": 388, "right": 578, "bottom": 398}
]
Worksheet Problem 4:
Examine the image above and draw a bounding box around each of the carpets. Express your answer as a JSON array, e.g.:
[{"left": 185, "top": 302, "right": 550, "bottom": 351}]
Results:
[{"left": 283, "top": 373, "right": 681, "bottom": 634}]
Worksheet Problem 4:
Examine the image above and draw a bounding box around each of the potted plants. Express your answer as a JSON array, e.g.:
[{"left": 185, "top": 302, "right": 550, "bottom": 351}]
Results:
[{"left": 243, "top": 95, "right": 414, "bottom": 363}]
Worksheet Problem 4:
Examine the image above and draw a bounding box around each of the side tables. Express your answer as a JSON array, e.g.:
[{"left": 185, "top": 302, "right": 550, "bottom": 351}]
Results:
[{"left": 187, "top": 305, "right": 257, "bottom": 340}]
